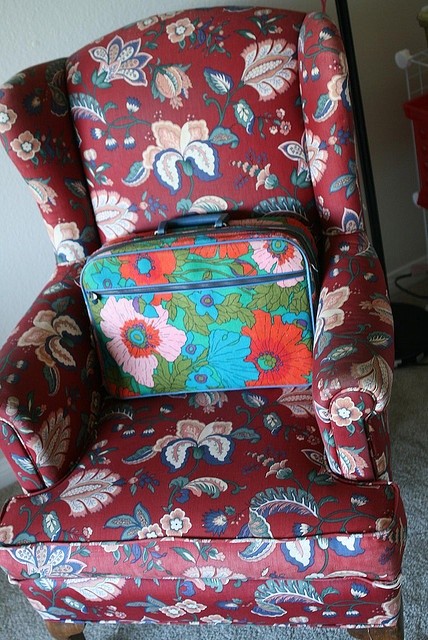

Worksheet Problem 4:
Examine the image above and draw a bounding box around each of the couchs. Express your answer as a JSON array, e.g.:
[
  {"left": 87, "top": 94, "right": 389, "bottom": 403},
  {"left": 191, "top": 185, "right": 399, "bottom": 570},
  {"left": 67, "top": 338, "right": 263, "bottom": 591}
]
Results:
[{"left": 0, "top": 5, "right": 404, "bottom": 640}]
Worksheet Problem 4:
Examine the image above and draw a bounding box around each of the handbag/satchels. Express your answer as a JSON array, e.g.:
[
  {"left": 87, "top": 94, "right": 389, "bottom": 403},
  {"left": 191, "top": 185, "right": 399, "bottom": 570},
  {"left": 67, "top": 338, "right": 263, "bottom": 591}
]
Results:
[{"left": 80, "top": 213, "right": 321, "bottom": 401}]
